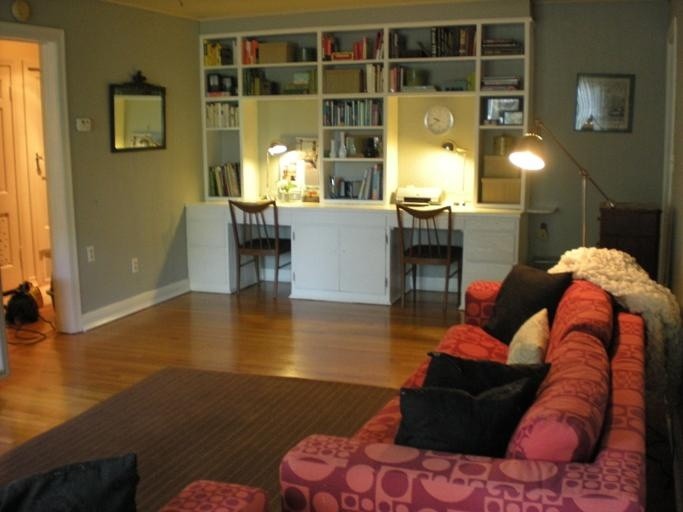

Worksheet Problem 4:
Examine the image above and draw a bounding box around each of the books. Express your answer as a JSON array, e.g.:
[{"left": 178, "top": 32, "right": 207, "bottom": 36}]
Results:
[
  {"left": 209, "top": 161, "right": 241, "bottom": 197},
  {"left": 430, "top": 26, "right": 476, "bottom": 57},
  {"left": 482, "top": 38, "right": 524, "bottom": 55},
  {"left": 481, "top": 71, "right": 522, "bottom": 91},
  {"left": 365, "top": 63, "right": 384, "bottom": 93},
  {"left": 204, "top": 68, "right": 238, "bottom": 97},
  {"left": 354, "top": 36, "right": 373, "bottom": 61},
  {"left": 206, "top": 102, "right": 239, "bottom": 128},
  {"left": 388, "top": 31, "right": 400, "bottom": 58},
  {"left": 400, "top": 85, "right": 440, "bottom": 92},
  {"left": 322, "top": 98, "right": 382, "bottom": 126},
  {"left": 203, "top": 42, "right": 233, "bottom": 66},
  {"left": 357, "top": 162, "right": 383, "bottom": 200},
  {"left": 243, "top": 69, "right": 272, "bottom": 95},
  {"left": 308, "top": 70, "right": 318, "bottom": 95},
  {"left": 372, "top": 31, "right": 384, "bottom": 60},
  {"left": 293, "top": 47, "right": 316, "bottom": 62},
  {"left": 241, "top": 38, "right": 259, "bottom": 64},
  {"left": 388, "top": 63, "right": 406, "bottom": 93},
  {"left": 282, "top": 87, "right": 308, "bottom": 95},
  {"left": 321, "top": 33, "right": 354, "bottom": 61}
]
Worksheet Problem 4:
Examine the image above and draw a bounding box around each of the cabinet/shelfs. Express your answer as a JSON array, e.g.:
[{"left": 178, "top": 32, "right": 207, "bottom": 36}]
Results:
[
  {"left": 459, "top": 210, "right": 529, "bottom": 310},
  {"left": 186, "top": 17, "right": 533, "bottom": 196},
  {"left": 184, "top": 201, "right": 237, "bottom": 294},
  {"left": 291, "top": 202, "right": 394, "bottom": 306}
]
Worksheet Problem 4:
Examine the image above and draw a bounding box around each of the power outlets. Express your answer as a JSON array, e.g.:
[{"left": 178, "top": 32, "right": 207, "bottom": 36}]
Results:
[
  {"left": 86, "top": 246, "right": 95, "bottom": 264},
  {"left": 75, "top": 118, "right": 92, "bottom": 132},
  {"left": 131, "top": 257, "right": 139, "bottom": 273}
]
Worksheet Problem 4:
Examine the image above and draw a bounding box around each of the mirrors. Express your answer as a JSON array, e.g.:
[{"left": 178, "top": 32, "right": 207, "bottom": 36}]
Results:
[{"left": 110, "top": 71, "right": 167, "bottom": 153}]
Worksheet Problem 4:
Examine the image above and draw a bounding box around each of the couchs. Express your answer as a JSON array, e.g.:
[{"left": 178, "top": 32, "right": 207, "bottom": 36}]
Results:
[{"left": 279, "top": 272, "right": 683, "bottom": 512}]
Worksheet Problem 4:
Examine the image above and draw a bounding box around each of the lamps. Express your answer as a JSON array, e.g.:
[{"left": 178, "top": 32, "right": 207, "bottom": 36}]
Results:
[
  {"left": 262, "top": 141, "right": 286, "bottom": 200},
  {"left": 509, "top": 117, "right": 612, "bottom": 239}
]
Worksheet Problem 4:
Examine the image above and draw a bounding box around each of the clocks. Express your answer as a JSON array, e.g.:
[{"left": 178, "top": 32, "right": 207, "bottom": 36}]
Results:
[
  {"left": 10, "top": 1, "right": 30, "bottom": 22},
  {"left": 425, "top": 104, "right": 453, "bottom": 135}
]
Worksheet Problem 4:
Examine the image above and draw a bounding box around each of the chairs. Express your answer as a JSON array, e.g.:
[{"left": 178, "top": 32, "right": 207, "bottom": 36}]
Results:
[
  {"left": 396, "top": 203, "right": 462, "bottom": 312},
  {"left": 228, "top": 199, "right": 291, "bottom": 300}
]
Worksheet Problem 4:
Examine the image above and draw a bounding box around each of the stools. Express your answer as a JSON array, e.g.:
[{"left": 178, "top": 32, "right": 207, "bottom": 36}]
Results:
[{"left": 162, "top": 480, "right": 268, "bottom": 512}]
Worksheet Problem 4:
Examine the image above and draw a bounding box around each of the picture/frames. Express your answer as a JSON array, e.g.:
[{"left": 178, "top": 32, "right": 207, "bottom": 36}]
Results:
[{"left": 572, "top": 71, "right": 635, "bottom": 134}]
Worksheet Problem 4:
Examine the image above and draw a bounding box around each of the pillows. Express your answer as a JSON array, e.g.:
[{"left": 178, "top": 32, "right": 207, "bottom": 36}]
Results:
[
  {"left": 507, "top": 308, "right": 551, "bottom": 365},
  {"left": 1, "top": 451, "right": 138, "bottom": 512},
  {"left": 482, "top": 264, "right": 572, "bottom": 346},
  {"left": 423, "top": 352, "right": 551, "bottom": 395},
  {"left": 394, "top": 378, "right": 536, "bottom": 458}
]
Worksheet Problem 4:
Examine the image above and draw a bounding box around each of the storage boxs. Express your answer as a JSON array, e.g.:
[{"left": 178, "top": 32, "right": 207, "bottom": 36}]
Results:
[
  {"left": 258, "top": 42, "right": 298, "bottom": 63},
  {"left": 324, "top": 70, "right": 364, "bottom": 91},
  {"left": 480, "top": 178, "right": 520, "bottom": 205},
  {"left": 482, "top": 154, "right": 520, "bottom": 178}
]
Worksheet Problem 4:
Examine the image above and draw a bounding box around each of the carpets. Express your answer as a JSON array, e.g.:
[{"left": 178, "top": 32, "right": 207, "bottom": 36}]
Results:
[{"left": 0, "top": 369, "right": 398, "bottom": 512}]
